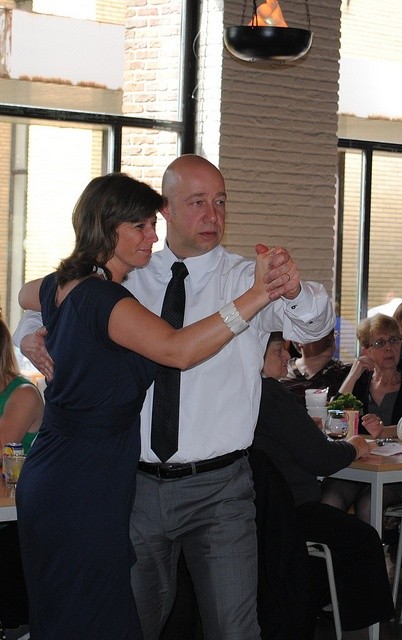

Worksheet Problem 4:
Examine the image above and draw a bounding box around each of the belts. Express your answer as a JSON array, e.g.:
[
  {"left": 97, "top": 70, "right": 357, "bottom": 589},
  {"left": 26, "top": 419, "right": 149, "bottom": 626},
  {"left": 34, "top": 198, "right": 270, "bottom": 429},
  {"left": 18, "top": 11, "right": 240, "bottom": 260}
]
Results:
[{"left": 138, "top": 450, "right": 244, "bottom": 479}]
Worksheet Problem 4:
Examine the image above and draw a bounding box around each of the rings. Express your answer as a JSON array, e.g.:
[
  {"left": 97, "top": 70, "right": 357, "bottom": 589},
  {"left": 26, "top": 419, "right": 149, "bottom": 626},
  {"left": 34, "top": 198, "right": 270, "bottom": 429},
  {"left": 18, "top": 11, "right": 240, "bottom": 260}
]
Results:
[
  {"left": 285, "top": 273, "right": 290, "bottom": 281},
  {"left": 379, "top": 420, "right": 383, "bottom": 425},
  {"left": 269, "top": 252, "right": 274, "bottom": 257}
]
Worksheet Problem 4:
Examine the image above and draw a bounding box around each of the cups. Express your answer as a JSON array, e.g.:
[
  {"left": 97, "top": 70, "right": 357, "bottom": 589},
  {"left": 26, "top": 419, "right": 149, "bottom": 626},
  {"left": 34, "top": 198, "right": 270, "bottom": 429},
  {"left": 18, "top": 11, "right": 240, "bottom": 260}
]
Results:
[
  {"left": 4, "top": 455, "right": 27, "bottom": 487},
  {"left": 305, "top": 389, "right": 327, "bottom": 431}
]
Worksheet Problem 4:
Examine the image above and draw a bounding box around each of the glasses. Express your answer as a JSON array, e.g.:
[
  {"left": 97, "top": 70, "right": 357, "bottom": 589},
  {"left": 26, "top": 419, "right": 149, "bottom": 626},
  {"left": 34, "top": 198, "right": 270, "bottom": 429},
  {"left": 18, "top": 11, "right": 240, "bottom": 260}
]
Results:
[{"left": 369, "top": 338, "right": 400, "bottom": 348}]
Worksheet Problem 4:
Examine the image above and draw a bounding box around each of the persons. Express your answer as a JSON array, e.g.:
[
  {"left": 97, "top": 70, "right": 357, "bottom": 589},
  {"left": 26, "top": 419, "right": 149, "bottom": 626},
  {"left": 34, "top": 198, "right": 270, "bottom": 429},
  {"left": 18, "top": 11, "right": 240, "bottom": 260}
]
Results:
[
  {"left": 11, "top": 155, "right": 336, "bottom": 640},
  {"left": 278, "top": 304, "right": 402, "bottom": 590},
  {"left": 246, "top": 331, "right": 396, "bottom": 640},
  {"left": 18, "top": 172, "right": 287, "bottom": 640},
  {"left": 0, "top": 319, "right": 45, "bottom": 457}
]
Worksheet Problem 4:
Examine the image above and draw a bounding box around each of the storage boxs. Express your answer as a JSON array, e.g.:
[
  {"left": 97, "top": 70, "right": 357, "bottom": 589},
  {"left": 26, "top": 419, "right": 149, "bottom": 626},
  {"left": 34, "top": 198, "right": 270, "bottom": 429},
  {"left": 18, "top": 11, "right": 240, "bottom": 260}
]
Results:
[{"left": 344, "top": 410, "right": 359, "bottom": 440}]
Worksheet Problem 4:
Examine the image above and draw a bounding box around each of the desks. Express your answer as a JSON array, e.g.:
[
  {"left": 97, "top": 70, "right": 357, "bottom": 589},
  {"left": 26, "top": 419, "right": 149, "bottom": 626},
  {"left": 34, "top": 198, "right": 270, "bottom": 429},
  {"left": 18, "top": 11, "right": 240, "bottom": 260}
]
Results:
[{"left": 328, "top": 435, "right": 402, "bottom": 640}]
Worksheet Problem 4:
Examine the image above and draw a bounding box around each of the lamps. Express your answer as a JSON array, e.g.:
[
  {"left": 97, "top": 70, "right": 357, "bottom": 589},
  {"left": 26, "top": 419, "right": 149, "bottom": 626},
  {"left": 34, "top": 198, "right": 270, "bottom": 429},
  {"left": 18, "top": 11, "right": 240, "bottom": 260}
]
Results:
[{"left": 223, "top": 0, "right": 314, "bottom": 62}]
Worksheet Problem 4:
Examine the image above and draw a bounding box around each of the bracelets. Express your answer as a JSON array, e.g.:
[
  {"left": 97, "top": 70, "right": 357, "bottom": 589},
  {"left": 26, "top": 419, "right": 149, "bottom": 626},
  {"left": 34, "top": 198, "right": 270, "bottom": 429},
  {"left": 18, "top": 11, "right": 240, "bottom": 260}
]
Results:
[{"left": 216, "top": 301, "right": 250, "bottom": 336}]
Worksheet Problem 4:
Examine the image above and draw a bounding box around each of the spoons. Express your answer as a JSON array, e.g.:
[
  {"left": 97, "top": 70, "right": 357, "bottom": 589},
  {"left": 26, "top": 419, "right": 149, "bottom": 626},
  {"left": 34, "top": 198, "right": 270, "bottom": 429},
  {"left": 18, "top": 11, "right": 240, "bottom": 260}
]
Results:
[{"left": 375, "top": 438, "right": 387, "bottom": 446}]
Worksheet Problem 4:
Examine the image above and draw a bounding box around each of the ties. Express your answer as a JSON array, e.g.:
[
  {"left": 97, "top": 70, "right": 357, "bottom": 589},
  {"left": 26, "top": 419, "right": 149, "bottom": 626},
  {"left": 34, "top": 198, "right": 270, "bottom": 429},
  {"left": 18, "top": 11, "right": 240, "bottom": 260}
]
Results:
[{"left": 150, "top": 262, "right": 190, "bottom": 462}]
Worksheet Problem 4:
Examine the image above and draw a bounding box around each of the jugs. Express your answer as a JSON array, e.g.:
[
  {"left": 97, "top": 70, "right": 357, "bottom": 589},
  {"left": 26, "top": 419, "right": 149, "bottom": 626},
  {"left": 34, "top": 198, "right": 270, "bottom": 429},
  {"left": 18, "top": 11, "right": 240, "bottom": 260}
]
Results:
[{"left": 323, "top": 409, "right": 350, "bottom": 440}]
